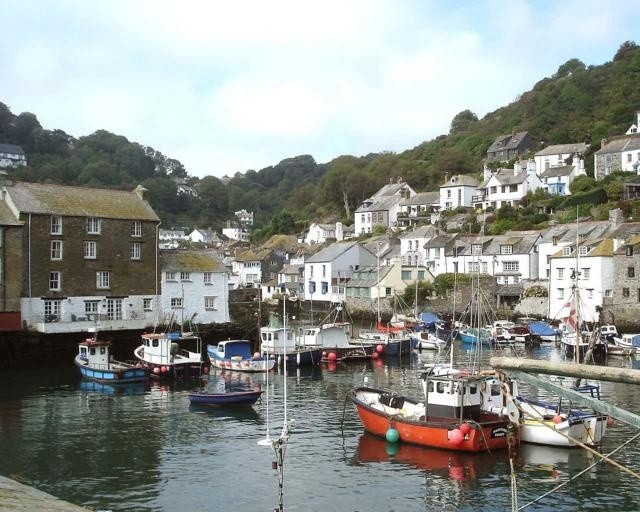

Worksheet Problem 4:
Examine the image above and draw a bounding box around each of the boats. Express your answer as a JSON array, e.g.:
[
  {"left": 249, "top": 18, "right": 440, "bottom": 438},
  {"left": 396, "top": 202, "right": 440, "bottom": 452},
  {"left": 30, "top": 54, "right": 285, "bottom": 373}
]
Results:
[
  {"left": 347, "top": 369, "right": 524, "bottom": 450},
  {"left": 206, "top": 339, "right": 276, "bottom": 372},
  {"left": 134, "top": 331, "right": 204, "bottom": 374},
  {"left": 75, "top": 338, "right": 146, "bottom": 382},
  {"left": 188, "top": 389, "right": 264, "bottom": 405}
]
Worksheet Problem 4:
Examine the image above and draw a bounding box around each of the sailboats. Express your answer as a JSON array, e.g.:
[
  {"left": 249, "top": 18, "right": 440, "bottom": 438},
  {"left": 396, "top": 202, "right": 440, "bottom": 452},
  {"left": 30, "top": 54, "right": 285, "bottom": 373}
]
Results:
[
  {"left": 419, "top": 290, "right": 608, "bottom": 446},
  {"left": 260, "top": 242, "right": 447, "bottom": 364},
  {"left": 452, "top": 202, "right": 640, "bottom": 365}
]
